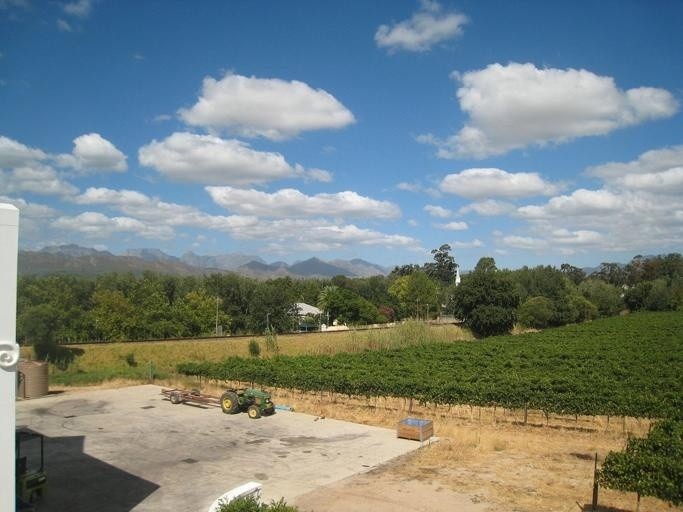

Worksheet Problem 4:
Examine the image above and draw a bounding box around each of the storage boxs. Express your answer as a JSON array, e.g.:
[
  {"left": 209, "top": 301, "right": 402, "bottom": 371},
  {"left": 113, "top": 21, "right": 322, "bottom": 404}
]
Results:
[{"left": 397, "top": 418, "right": 434, "bottom": 442}]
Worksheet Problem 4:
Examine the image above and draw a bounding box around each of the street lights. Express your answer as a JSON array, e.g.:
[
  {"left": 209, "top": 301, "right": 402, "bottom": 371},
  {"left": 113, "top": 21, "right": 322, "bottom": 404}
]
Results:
[{"left": 266, "top": 312, "right": 272, "bottom": 328}]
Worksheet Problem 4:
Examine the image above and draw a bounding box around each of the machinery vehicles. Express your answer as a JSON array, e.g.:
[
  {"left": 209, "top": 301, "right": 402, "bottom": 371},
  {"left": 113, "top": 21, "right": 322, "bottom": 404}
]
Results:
[{"left": 218, "top": 385, "right": 275, "bottom": 418}]
